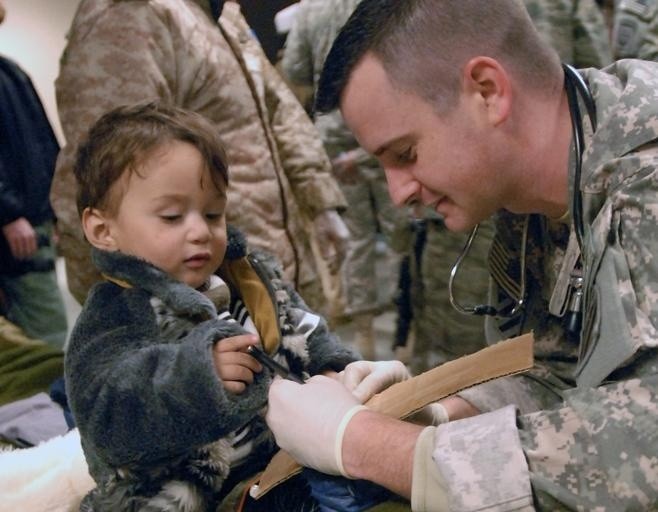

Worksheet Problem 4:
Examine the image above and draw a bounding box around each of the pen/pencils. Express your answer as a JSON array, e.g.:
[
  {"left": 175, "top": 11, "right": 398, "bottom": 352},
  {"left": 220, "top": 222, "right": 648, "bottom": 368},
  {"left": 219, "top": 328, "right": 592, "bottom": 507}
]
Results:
[{"left": 246, "top": 344, "right": 306, "bottom": 385}]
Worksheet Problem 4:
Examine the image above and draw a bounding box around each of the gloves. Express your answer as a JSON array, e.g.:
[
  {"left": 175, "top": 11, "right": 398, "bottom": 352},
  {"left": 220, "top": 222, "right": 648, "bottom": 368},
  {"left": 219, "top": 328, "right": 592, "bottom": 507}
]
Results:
[
  {"left": 266, "top": 373, "right": 368, "bottom": 482},
  {"left": 337, "top": 358, "right": 448, "bottom": 427}
]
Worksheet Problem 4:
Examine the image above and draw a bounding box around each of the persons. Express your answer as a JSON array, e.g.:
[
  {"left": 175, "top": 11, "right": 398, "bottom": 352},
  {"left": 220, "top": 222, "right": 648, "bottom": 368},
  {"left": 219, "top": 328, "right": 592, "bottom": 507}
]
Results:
[
  {"left": 0, "top": 1, "right": 656, "bottom": 440},
  {"left": 57, "top": 99, "right": 384, "bottom": 512},
  {"left": 264, "top": 0, "right": 657, "bottom": 512}
]
